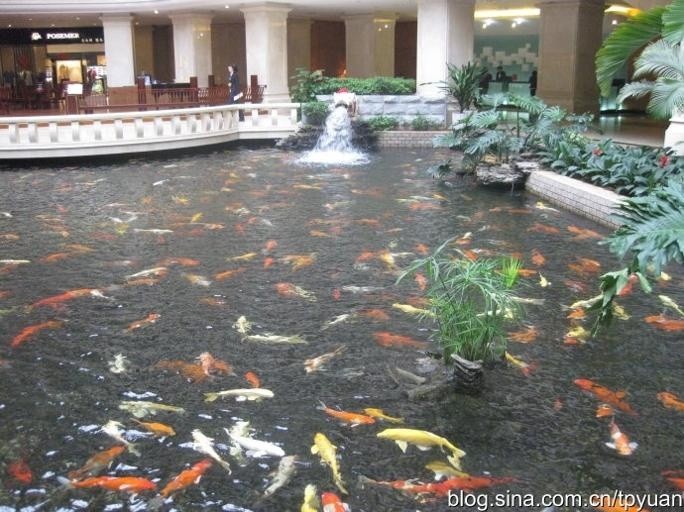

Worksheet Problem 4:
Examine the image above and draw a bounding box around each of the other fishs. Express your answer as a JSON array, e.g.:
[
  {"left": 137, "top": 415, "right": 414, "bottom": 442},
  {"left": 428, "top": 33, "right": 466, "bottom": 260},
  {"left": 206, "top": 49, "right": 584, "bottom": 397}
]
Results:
[{"left": 0, "top": 150, "right": 684, "bottom": 512}]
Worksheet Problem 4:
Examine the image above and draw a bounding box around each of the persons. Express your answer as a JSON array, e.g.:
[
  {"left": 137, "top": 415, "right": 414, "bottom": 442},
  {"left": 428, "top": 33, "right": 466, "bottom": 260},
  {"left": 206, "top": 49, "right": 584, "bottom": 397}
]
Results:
[
  {"left": 479, "top": 74, "right": 489, "bottom": 97},
  {"left": 495, "top": 65, "right": 505, "bottom": 80},
  {"left": 87, "top": 68, "right": 97, "bottom": 86},
  {"left": 223, "top": 63, "right": 245, "bottom": 122},
  {"left": 527, "top": 70, "right": 537, "bottom": 97}
]
